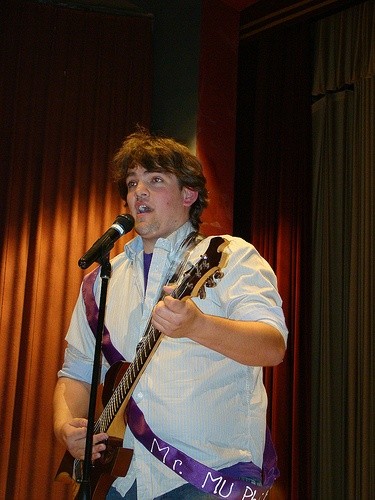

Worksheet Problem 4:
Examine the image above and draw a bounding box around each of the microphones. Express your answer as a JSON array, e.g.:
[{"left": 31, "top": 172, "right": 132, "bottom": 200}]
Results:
[{"left": 78, "top": 214, "right": 135, "bottom": 270}]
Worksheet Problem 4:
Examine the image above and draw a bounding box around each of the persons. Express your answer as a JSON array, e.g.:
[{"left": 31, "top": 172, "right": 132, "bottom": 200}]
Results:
[{"left": 52, "top": 132, "right": 290, "bottom": 500}]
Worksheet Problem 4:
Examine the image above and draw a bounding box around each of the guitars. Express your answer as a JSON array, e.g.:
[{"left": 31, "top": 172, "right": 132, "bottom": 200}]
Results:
[{"left": 50, "top": 236, "right": 230, "bottom": 500}]
